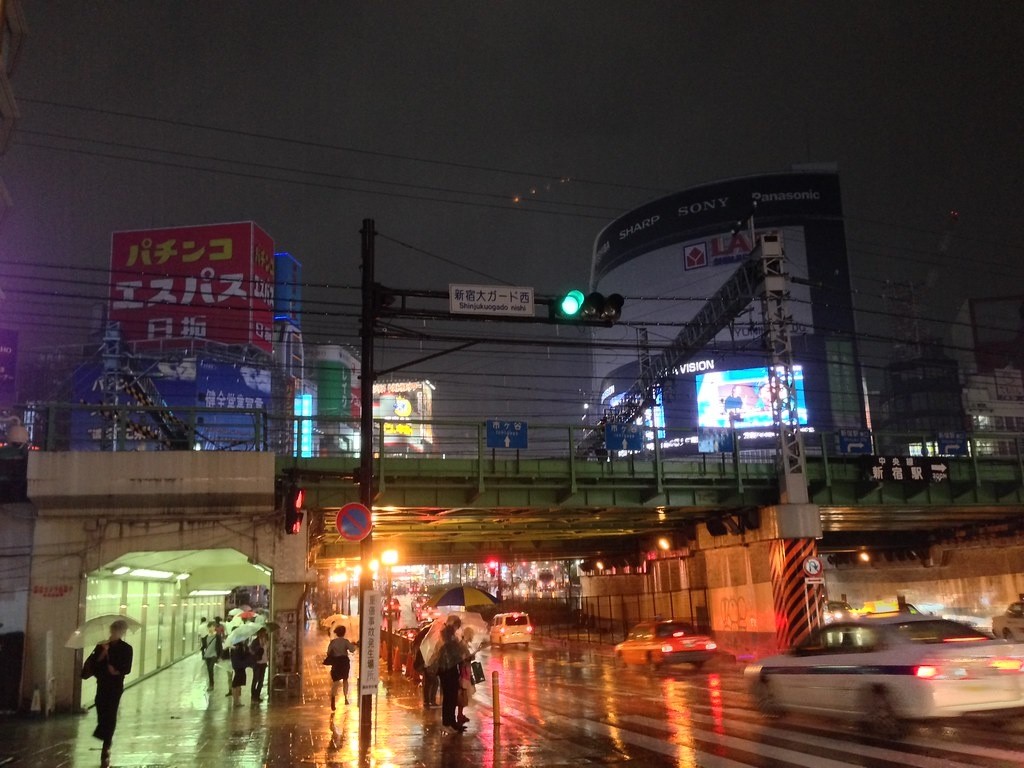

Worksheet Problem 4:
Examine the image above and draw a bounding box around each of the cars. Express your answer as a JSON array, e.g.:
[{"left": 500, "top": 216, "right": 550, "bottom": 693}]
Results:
[
  {"left": 380, "top": 578, "right": 570, "bottom": 640},
  {"left": 828, "top": 600, "right": 857, "bottom": 621},
  {"left": 991, "top": 600, "right": 1023, "bottom": 643},
  {"left": 859, "top": 603, "right": 945, "bottom": 643},
  {"left": 614, "top": 619, "right": 718, "bottom": 672},
  {"left": 743, "top": 615, "right": 1024, "bottom": 735}
]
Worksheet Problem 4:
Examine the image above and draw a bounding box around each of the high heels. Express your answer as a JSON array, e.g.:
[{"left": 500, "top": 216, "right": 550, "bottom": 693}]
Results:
[{"left": 441, "top": 725, "right": 457, "bottom": 736}]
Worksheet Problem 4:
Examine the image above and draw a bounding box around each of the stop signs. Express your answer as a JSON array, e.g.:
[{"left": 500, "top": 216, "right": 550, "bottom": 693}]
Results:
[{"left": 335, "top": 502, "right": 373, "bottom": 542}]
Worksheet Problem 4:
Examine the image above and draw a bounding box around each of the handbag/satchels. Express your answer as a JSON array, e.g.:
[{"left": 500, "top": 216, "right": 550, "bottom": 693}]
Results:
[
  {"left": 323, "top": 653, "right": 332, "bottom": 665},
  {"left": 79, "top": 643, "right": 103, "bottom": 679},
  {"left": 470, "top": 661, "right": 485, "bottom": 684}
]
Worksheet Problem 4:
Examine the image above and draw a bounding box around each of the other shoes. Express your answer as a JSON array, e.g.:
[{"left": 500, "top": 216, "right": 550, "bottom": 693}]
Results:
[
  {"left": 233, "top": 701, "right": 245, "bottom": 706},
  {"left": 331, "top": 702, "right": 336, "bottom": 711},
  {"left": 225, "top": 690, "right": 232, "bottom": 696},
  {"left": 207, "top": 686, "right": 214, "bottom": 691},
  {"left": 345, "top": 700, "right": 350, "bottom": 704},
  {"left": 104, "top": 751, "right": 111, "bottom": 765},
  {"left": 457, "top": 715, "right": 470, "bottom": 722},
  {"left": 252, "top": 697, "right": 263, "bottom": 702},
  {"left": 424, "top": 702, "right": 441, "bottom": 708}
]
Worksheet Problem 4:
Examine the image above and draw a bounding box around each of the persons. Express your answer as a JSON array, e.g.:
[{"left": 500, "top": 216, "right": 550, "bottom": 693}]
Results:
[
  {"left": 723, "top": 373, "right": 792, "bottom": 415},
  {"left": 198, "top": 616, "right": 270, "bottom": 709},
  {"left": 324, "top": 624, "right": 359, "bottom": 711},
  {"left": 84, "top": 618, "right": 132, "bottom": 768},
  {"left": 411, "top": 618, "right": 491, "bottom": 730}
]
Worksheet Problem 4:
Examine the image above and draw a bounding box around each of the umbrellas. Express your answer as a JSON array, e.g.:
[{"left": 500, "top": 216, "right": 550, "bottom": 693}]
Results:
[
  {"left": 62, "top": 613, "right": 145, "bottom": 656},
  {"left": 421, "top": 585, "right": 502, "bottom": 630},
  {"left": 322, "top": 613, "right": 361, "bottom": 641},
  {"left": 419, "top": 610, "right": 487, "bottom": 670},
  {"left": 217, "top": 602, "right": 270, "bottom": 650}
]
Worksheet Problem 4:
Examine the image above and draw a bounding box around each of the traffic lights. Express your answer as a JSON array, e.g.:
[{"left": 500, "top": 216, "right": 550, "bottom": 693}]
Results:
[
  {"left": 285, "top": 486, "right": 305, "bottom": 535},
  {"left": 547, "top": 290, "right": 625, "bottom": 323}
]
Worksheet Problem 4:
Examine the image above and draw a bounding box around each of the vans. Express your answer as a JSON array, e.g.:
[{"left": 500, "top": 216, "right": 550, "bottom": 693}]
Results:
[{"left": 489, "top": 612, "right": 533, "bottom": 648}]
